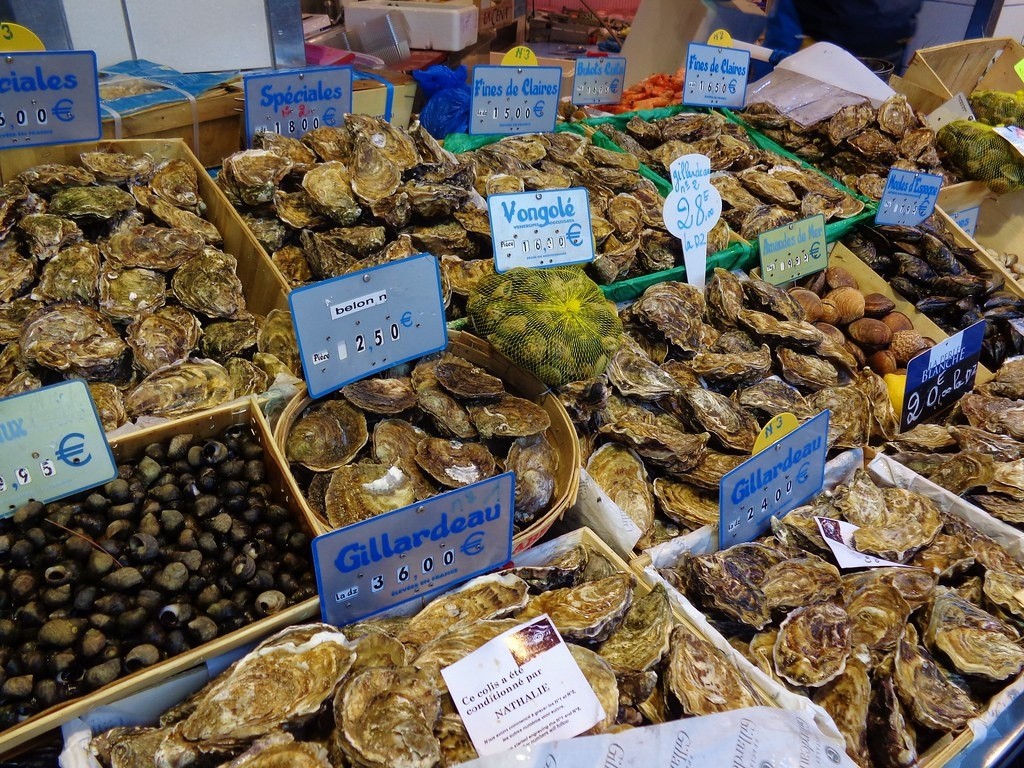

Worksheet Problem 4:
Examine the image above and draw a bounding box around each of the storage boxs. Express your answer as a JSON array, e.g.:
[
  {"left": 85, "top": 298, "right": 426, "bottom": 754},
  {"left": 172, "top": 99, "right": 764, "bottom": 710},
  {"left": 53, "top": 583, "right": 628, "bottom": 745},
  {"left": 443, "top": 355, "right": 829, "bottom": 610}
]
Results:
[{"left": 1, "top": 7, "right": 1023, "bottom": 768}]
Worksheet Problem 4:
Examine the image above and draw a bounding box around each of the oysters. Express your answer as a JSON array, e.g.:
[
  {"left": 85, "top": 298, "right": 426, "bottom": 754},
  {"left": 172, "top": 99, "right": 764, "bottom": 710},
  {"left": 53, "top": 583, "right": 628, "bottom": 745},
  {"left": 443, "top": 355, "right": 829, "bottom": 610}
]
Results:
[{"left": 0, "top": 95, "right": 1024, "bottom": 768}]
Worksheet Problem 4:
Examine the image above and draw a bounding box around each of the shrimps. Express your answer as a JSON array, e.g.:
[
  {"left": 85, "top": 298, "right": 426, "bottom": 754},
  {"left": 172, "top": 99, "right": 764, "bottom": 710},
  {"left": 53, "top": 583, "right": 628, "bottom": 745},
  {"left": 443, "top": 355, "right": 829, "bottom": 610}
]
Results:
[{"left": 587, "top": 67, "right": 686, "bottom": 114}]
